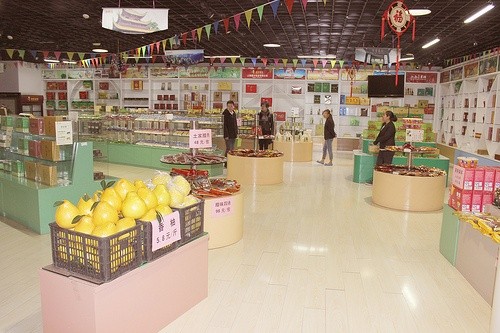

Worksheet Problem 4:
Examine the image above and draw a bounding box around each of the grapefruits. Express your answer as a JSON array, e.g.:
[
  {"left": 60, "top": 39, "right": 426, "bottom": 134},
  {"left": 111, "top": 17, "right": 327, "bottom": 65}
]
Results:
[
  {"left": 151, "top": 172, "right": 201, "bottom": 238},
  {"left": 55, "top": 178, "right": 173, "bottom": 273}
]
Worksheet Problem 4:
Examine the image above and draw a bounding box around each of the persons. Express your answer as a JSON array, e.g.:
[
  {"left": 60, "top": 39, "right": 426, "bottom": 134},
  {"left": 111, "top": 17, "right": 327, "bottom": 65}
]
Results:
[
  {"left": 255, "top": 102, "right": 273, "bottom": 150},
  {"left": 222, "top": 101, "right": 238, "bottom": 168},
  {"left": 316, "top": 109, "right": 337, "bottom": 166},
  {"left": 365, "top": 111, "right": 397, "bottom": 185}
]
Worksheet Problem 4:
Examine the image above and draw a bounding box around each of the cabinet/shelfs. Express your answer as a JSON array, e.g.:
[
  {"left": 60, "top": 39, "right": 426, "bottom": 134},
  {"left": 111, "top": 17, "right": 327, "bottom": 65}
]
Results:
[
  {"left": 227, "top": 119, "right": 283, "bottom": 185},
  {"left": 0, "top": 114, "right": 122, "bottom": 235},
  {"left": 372, "top": 144, "right": 447, "bottom": 212},
  {"left": 438, "top": 207, "right": 500, "bottom": 306},
  {"left": 78, "top": 111, "right": 225, "bottom": 178},
  {"left": 42, "top": 55, "right": 500, "bottom": 161},
  {"left": 273, "top": 115, "right": 313, "bottom": 162},
  {"left": 159, "top": 130, "right": 244, "bottom": 250}
]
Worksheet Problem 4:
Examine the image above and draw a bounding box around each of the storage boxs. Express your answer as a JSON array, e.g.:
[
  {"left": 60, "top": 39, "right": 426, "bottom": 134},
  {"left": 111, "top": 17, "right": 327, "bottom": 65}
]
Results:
[
  {"left": 0, "top": 115, "right": 68, "bottom": 135},
  {"left": 49, "top": 198, "right": 204, "bottom": 280},
  {"left": 450, "top": 166, "right": 500, "bottom": 212},
  {"left": 361, "top": 105, "right": 434, "bottom": 141},
  {"left": 0, "top": 139, "right": 62, "bottom": 186}
]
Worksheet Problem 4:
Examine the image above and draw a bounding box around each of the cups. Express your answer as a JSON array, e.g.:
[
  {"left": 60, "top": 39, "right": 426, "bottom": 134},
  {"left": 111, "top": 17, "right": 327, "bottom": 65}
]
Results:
[{"left": 457, "top": 156, "right": 478, "bottom": 168}]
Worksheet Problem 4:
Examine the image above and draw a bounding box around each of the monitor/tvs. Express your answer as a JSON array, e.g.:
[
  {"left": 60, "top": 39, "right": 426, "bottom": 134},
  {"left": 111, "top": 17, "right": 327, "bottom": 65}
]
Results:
[{"left": 368, "top": 75, "right": 404, "bottom": 98}]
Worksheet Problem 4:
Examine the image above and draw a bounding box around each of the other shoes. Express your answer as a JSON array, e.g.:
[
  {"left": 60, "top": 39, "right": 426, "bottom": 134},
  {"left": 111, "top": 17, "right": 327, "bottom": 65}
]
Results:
[
  {"left": 324, "top": 163, "right": 333, "bottom": 166},
  {"left": 317, "top": 161, "right": 324, "bottom": 164},
  {"left": 365, "top": 181, "right": 372, "bottom": 186}
]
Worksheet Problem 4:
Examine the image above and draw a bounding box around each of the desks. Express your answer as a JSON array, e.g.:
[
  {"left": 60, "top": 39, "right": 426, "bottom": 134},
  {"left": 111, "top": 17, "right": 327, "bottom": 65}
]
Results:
[{"left": 42, "top": 236, "right": 211, "bottom": 333}]
